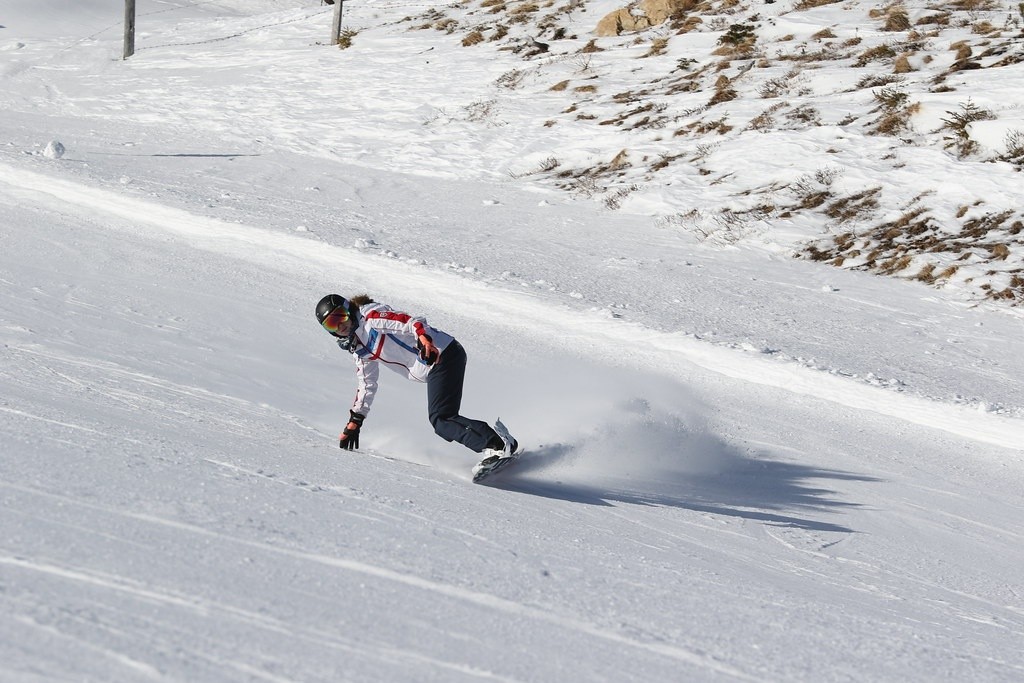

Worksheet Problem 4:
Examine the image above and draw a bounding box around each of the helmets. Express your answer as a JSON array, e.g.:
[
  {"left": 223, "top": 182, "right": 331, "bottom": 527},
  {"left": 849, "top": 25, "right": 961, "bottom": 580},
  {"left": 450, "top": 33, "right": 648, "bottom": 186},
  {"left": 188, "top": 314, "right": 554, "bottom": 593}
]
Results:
[{"left": 315, "top": 294, "right": 356, "bottom": 337}]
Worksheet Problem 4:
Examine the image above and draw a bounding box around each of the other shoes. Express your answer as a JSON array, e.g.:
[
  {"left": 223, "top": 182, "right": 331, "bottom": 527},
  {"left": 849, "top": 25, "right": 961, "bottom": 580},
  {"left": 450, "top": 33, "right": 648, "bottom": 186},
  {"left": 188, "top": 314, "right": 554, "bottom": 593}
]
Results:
[
  {"left": 481, "top": 436, "right": 518, "bottom": 466},
  {"left": 472, "top": 461, "right": 485, "bottom": 475}
]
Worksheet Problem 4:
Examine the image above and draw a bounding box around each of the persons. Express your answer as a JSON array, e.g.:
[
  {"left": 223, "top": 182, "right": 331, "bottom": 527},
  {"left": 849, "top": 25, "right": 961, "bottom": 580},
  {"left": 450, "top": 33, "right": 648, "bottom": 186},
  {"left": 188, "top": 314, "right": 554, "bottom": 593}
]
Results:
[{"left": 314, "top": 293, "right": 519, "bottom": 475}]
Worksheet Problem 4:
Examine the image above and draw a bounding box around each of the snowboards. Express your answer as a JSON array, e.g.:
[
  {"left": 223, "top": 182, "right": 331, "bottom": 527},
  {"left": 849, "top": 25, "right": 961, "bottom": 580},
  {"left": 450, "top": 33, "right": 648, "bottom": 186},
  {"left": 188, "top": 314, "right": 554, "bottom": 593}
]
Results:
[{"left": 471, "top": 433, "right": 526, "bottom": 484}]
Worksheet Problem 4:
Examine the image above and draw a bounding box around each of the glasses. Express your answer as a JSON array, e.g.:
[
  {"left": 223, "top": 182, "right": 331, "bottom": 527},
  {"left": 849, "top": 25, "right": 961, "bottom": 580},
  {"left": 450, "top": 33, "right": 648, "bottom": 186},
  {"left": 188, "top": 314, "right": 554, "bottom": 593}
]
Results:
[{"left": 321, "top": 300, "right": 351, "bottom": 333}]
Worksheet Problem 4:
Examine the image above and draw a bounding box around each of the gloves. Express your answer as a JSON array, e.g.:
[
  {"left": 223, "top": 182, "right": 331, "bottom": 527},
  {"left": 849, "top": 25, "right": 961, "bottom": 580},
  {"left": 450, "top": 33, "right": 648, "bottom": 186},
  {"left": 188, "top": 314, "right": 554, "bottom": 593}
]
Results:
[
  {"left": 417, "top": 334, "right": 440, "bottom": 370},
  {"left": 339, "top": 408, "right": 366, "bottom": 451}
]
